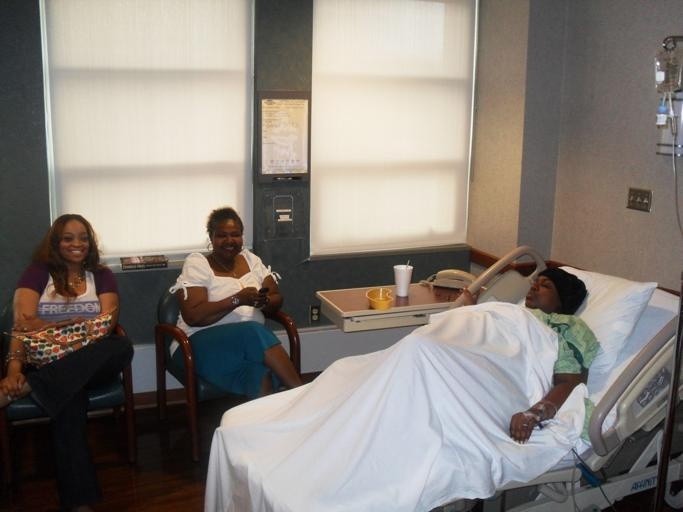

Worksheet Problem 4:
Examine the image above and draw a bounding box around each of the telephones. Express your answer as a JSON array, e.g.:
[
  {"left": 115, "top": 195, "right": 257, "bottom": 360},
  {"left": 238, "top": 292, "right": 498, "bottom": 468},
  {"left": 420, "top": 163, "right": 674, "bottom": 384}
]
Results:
[{"left": 433, "top": 269, "right": 477, "bottom": 289}]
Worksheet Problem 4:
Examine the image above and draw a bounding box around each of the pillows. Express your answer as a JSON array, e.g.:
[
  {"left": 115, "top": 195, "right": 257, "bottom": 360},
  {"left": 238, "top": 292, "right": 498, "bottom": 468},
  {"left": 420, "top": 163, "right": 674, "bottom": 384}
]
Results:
[{"left": 552, "top": 260, "right": 662, "bottom": 380}]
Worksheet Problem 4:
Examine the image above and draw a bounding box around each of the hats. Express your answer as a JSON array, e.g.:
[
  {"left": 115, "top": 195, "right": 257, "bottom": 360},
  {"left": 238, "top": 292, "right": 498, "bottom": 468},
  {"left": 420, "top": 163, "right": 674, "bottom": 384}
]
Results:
[{"left": 538, "top": 267, "right": 586, "bottom": 315}]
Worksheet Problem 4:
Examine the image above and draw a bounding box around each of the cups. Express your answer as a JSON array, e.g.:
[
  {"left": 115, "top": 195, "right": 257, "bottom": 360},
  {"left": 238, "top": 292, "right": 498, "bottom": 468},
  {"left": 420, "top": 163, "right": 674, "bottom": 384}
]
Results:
[{"left": 392, "top": 263, "right": 414, "bottom": 298}]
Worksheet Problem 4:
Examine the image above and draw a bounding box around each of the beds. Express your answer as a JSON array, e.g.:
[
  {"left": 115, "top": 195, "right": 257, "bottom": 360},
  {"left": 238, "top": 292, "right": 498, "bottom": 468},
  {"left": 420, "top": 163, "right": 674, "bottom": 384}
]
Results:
[{"left": 192, "top": 241, "right": 683, "bottom": 510}]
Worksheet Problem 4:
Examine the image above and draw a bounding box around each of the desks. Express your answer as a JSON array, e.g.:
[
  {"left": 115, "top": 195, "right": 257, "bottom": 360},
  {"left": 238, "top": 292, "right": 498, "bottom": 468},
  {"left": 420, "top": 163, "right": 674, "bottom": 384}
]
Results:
[{"left": 315, "top": 281, "right": 487, "bottom": 333}]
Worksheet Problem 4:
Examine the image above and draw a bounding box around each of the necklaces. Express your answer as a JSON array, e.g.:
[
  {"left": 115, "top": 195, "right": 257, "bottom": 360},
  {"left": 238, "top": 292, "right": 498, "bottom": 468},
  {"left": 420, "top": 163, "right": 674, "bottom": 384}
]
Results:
[
  {"left": 214, "top": 252, "right": 237, "bottom": 277},
  {"left": 67, "top": 269, "right": 83, "bottom": 289}
]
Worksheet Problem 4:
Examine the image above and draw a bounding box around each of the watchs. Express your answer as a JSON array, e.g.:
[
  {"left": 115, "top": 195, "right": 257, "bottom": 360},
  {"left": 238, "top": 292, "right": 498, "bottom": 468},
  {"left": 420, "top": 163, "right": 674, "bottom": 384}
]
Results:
[{"left": 231, "top": 294, "right": 240, "bottom": 309}]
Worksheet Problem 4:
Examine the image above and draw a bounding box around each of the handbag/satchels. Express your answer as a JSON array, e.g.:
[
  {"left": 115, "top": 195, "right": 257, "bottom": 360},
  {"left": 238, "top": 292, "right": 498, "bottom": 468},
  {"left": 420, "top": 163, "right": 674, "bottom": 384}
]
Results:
[{"left": 3, "top": 306, "right": 118, "bottom": 371}]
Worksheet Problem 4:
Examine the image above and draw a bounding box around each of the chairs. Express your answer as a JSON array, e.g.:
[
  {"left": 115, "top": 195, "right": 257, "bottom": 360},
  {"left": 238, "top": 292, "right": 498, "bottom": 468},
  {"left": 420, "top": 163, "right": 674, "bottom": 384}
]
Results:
[
  {"left": 0, "top": 294, "right": 150, "bottom": 487},
  {"left": 150, "top": 273, "right": 303, "bottom": 468}
]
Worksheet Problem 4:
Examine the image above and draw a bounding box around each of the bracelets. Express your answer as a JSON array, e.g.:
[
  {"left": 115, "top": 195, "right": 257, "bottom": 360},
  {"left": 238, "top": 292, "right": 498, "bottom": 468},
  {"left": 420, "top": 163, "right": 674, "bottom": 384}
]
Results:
[{"left": 5, "top": 351, "right": 26, "bottom": 367}]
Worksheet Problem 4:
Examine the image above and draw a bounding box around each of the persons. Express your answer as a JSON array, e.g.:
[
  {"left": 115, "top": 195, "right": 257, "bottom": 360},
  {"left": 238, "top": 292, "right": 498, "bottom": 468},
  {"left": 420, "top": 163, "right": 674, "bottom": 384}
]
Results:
[
  {"left": 462, "top": 268, "right": 599, "bottom": 444},
  {"left": 164, "top": 207, "right": 302, "bottom": 400},
  {"left": 0, "top": 214, "right": 135, "bottom": 512}
]
